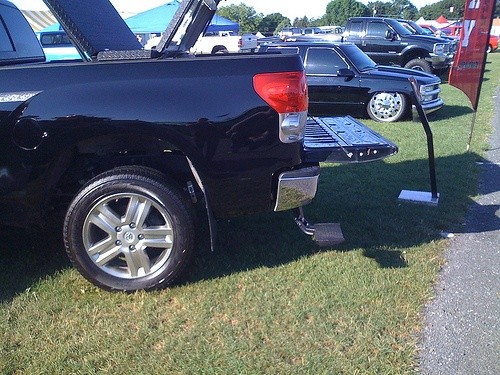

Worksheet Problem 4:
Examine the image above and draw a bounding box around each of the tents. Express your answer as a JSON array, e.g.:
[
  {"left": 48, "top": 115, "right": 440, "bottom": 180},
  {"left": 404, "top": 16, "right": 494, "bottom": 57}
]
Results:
[
  {"left": 414, "top": 15, "right": 455, "bottom": 24},
  {"left": 35, "top": 0, "right": 240, "bottom": 63}
]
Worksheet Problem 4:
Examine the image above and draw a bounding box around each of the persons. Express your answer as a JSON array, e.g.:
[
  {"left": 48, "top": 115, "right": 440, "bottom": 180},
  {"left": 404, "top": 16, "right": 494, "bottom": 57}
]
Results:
[{"left": 436, "top": 26, "right": 451, "bottom": 38}]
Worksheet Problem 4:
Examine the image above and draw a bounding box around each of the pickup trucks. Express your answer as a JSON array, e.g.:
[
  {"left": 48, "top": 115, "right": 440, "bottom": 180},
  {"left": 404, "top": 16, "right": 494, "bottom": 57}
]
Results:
[
  {"left": 258, "top": 17, "right": 454, "bottom": 75},
  {"left": 257, "top": 40, "right": 445, "bottom": 122},
  {"left": 1, "top": 0, "right": 399, "bottom": 294},
  {"left": 144, "top": 28, "right": 243, "bottom": 53}
]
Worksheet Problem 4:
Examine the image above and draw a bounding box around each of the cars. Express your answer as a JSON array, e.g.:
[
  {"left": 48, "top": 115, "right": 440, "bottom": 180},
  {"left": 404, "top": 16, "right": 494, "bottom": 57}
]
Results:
[{"left": 396, "top": 18, "right": 500, "bottom": 58}]
